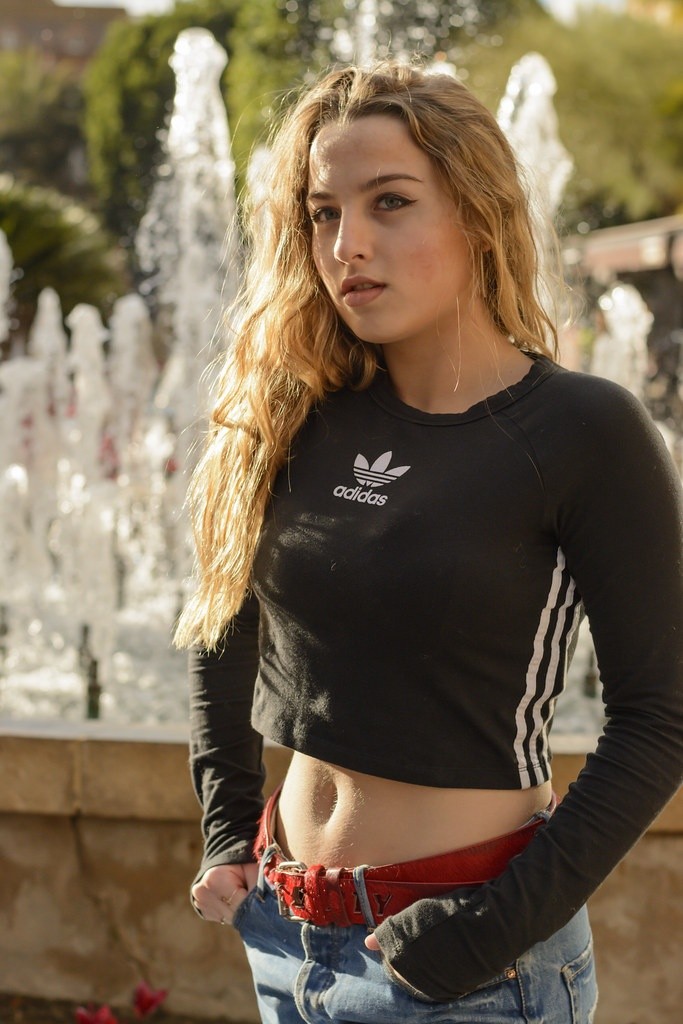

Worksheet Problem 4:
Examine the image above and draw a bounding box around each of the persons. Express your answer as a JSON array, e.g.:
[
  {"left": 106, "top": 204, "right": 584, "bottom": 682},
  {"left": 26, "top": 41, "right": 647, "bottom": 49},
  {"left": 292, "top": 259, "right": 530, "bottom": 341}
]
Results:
[{"left": 173, "top": 68, "right": 682, "bottom": 1024}]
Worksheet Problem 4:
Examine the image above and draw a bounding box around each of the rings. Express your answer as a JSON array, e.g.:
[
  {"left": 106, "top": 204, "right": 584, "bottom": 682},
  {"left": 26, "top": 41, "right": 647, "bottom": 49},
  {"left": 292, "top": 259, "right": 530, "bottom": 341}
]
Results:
[
  {"left": 222, "top": 887, "right": 239, "bottom": 905},
  {"left": 220, "top": 917, "right": 226, "bottom": 924}
]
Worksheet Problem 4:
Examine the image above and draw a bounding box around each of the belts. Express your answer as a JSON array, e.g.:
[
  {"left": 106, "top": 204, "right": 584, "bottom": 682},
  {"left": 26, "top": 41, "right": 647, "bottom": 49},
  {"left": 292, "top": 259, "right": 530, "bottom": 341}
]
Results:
[{"left": 254, "top": 780, "right": 556, "bottom": 927}]
